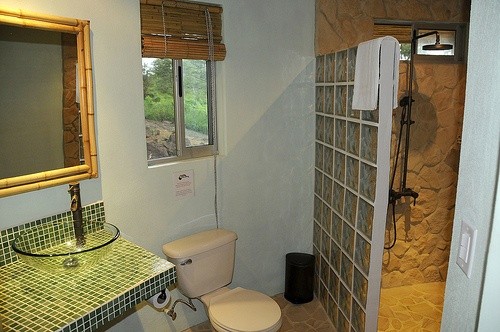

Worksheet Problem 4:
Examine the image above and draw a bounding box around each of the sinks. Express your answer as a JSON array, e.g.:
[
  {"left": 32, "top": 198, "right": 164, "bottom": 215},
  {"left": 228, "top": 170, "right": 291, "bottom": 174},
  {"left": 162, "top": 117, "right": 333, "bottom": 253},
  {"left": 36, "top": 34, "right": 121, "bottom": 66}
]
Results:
[{"left": 10, "top": 219, "right": 121, "bottom": 274}]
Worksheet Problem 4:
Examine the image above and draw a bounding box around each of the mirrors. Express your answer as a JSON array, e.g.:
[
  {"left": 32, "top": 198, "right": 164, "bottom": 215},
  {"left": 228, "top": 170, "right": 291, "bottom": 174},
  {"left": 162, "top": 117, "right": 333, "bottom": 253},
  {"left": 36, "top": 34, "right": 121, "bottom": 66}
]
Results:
[{"left": 0, "top": 3, "right": 99, "bottom": 199}]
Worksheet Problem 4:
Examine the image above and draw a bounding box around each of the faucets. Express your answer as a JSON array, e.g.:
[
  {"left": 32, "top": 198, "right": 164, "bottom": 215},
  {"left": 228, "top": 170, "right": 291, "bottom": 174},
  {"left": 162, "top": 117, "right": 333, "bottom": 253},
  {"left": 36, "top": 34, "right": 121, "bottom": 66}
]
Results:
[{"left": 67, "top": 181, "right": 86, "bottom": 245}]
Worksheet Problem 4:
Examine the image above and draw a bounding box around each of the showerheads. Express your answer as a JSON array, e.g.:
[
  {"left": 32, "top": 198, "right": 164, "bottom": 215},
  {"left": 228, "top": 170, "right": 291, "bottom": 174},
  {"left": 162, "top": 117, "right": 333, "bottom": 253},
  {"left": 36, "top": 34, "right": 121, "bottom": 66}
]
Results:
[
  {"left": 400, "top": 97, "right": 410, "bottom": 126},
  {"left": 422, "top": 30, "right": 454, "bottom": 52}
]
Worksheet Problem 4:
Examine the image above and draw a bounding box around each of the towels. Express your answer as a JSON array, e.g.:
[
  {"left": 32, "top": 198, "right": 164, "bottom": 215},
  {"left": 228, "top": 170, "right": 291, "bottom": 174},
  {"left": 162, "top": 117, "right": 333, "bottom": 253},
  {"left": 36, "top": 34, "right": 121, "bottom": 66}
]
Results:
[{"left": 352, "top": 35, "right": 401, "bottom": 110}]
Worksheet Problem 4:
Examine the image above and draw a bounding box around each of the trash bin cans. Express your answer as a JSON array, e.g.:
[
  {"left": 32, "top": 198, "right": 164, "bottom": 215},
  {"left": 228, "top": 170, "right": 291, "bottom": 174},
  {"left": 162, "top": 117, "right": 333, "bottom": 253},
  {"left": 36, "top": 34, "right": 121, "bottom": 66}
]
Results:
[{"left": 282, "top": 251, "right": 316, "bottom": 304}]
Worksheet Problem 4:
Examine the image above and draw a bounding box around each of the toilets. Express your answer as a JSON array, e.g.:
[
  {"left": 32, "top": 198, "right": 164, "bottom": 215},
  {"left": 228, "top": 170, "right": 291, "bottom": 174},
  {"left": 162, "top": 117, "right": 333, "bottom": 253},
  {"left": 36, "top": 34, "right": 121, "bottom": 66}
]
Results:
[{"left": 161, "top": 228, "right": 282, "bottom": 332}]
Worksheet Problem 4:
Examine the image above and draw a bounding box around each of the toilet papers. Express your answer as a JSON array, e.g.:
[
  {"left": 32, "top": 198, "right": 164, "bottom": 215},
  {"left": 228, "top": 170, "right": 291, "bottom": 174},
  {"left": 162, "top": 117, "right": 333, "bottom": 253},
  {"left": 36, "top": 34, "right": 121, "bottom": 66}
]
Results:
[{"left": 146, "top": 288, "right": 171, "bottom": 310}]
